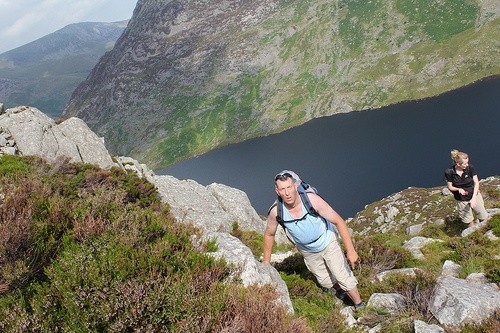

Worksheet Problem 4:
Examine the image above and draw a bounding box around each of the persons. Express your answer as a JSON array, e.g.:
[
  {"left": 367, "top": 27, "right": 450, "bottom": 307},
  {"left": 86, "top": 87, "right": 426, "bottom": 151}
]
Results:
[
  {"left": 262, "top": 173, "right": 365, "bottom": 308},
  {"left": 444, "top": 149, "right": 489, "bottom": 227}
]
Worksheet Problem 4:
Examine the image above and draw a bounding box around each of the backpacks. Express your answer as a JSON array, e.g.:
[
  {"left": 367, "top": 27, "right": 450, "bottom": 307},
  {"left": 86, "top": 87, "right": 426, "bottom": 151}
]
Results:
[{"left": 274, "top": 169, "right": 318, "bottom": 194}]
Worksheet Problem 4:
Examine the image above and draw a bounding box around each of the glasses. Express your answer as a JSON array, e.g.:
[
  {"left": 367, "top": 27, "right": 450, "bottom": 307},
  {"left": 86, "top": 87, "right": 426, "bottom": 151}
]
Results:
[{"left": 276, "top": 173, "right": 291, "bottom": 179}]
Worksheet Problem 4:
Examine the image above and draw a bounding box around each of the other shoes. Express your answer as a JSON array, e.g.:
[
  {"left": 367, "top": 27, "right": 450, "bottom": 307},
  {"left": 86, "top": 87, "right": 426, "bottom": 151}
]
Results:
[
  {"left": 328, "top": 288, "right": 344, "bottom": 307},
  {"left": 355, "top": 304, "right": 366, "bottom": 311}
]
